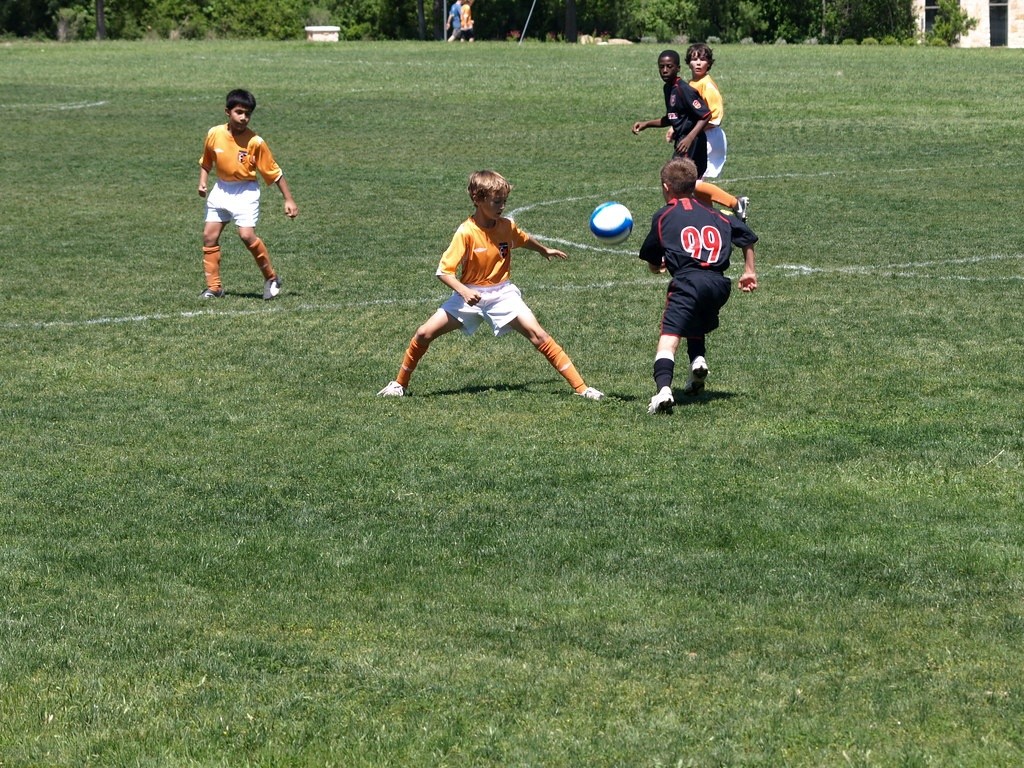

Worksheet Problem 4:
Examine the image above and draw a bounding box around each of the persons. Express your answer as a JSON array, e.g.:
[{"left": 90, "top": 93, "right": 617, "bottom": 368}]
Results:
[
  {"left": 639, "top": 158, "right": 758, "bottom": 417},
  {"left": 634, "top": 43, "right": 748, "bottom": 223},
  {"left": 192, "top": 87, "right": 298, "bottom": 302},
  {"left": 377, "top": 170, "right": 602, "bottom": 401},
  {"left": 446, "top": 0, "right": 475, "bottom": 42}
]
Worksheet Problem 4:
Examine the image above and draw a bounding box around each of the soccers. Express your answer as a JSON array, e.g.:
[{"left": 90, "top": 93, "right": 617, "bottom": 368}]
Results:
[{"left": 590, "top": 199, "right": 634, "bottom": 244}]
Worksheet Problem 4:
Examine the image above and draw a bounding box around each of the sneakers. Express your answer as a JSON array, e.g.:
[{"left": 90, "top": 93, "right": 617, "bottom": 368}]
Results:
[
  {"left": 574, "top": 386, "right": 604, "bottom": 402},
  {"left": 733, "top": 196, "right": 750, "bottom": 226},
  {"left": 647, "top": 386, "right": 674, "bottom": 416},
  {"left": 377, "top": 381, "right": 405, "bottom": 397},
  {"left": 198, "top": 288, "right": 225, "bottom": 300},
  {"left": 263, "top": 275, "right": 281, "bottom": 300},
  {"left": 684, "top": 356, "right": 708, "bottom": 395}
]
